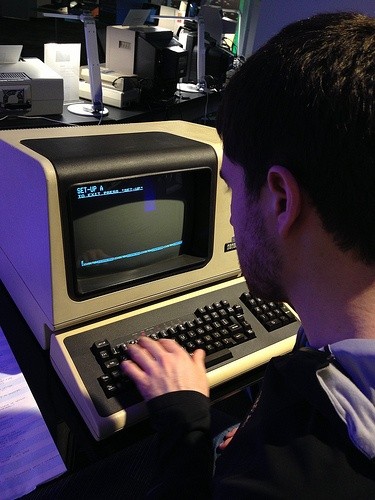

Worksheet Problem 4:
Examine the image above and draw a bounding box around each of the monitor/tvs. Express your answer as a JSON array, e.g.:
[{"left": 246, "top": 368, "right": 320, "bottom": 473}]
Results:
[
  {"left": 106, "top": 25, "right": 188, "bottom": 99},
  {"left": 0, "top": 120, "right": 242, "bottom": 351},
  {"left": 178, "top": 29, "right": 234, "bottom": 93}
]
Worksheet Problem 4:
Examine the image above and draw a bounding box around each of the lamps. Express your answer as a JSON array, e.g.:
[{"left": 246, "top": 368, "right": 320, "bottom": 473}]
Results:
[
  {"left": 42, "top": 12, "right": 109, "bottom": 117},
  {"left": 152, "top": 15, "right": 206, "bottom": 93}
]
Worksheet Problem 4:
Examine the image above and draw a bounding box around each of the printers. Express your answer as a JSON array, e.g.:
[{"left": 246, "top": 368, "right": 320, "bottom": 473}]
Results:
[{"left": 0, "top": 45, "right": 63, "bottom": 117}]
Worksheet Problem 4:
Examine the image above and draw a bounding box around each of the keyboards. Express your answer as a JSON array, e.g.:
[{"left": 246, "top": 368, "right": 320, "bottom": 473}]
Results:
[{"left": 49, "top": 275, "right": 302, "bottom": 441}]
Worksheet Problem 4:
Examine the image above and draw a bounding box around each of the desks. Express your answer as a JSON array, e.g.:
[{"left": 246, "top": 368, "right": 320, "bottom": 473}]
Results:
[{"left": 0, "top": 62, "right": 223, "bottom": 130}]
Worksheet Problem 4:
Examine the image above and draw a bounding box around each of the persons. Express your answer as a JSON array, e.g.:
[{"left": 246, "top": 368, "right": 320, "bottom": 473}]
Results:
[{"left": 112, "top": 11, "right": 375, "bottom": 500}]
